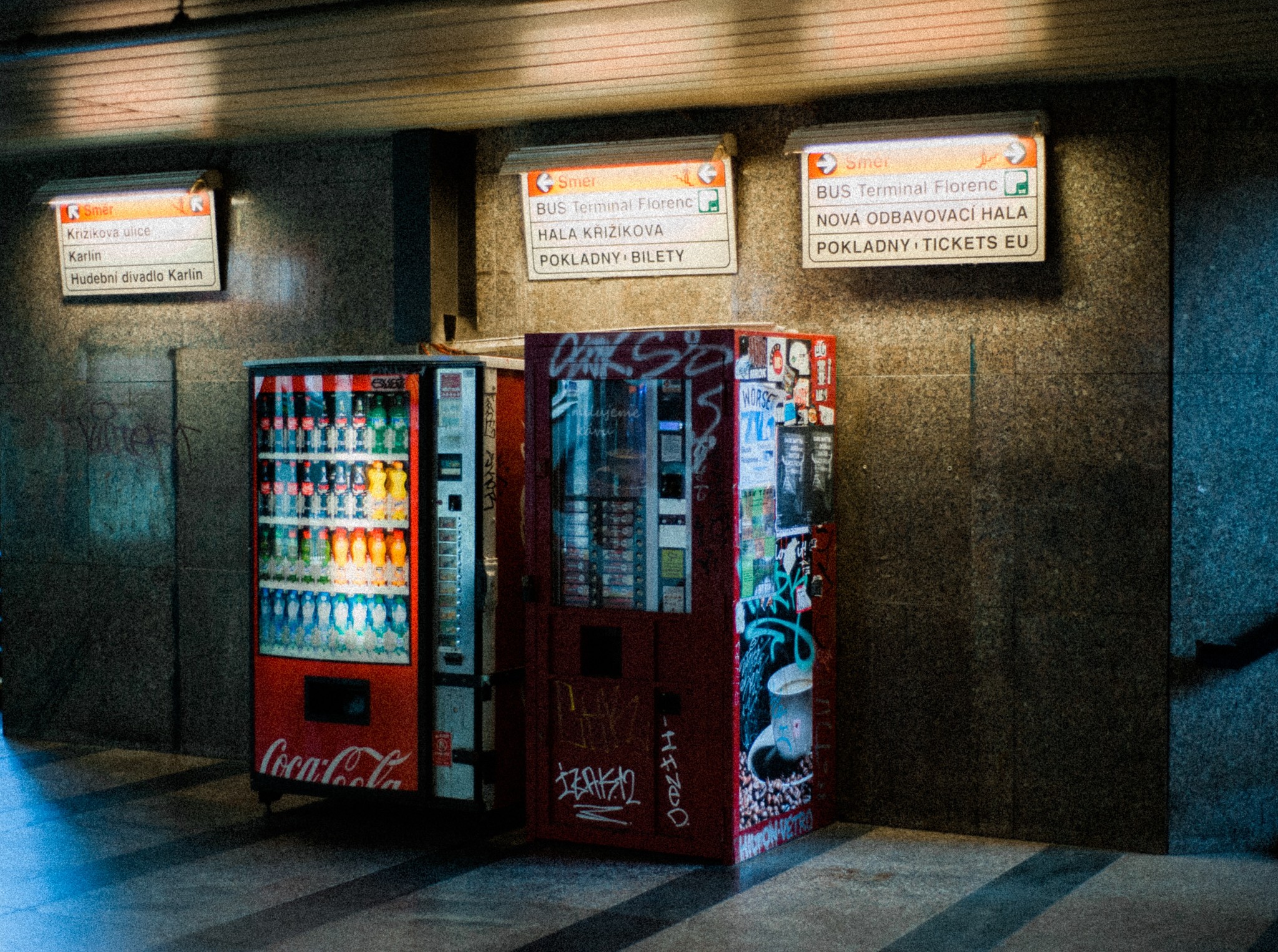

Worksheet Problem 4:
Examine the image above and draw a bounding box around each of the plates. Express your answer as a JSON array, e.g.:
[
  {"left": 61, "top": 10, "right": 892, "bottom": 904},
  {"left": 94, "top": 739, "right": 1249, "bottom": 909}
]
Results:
[
  {"left": 747, "top": 724, "right": 813, "bottom": 787},
  {"left": 593, "top": 478, "right": 647, "bottom": 497}
]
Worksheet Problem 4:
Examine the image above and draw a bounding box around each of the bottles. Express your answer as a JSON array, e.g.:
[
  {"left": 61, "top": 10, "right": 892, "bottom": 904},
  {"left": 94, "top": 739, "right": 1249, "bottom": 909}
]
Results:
[
  {"left": 259, "top": 460, "right": 407, "bottom": 521},
  {"left": 259, "top": 393, "right": 408, "bottom": 454},
  {"left": 260, "top": 526, "right": 407, "bottom": 586},
  {"left": 258, "top": 589, "right": 408, "bottom": 656}
]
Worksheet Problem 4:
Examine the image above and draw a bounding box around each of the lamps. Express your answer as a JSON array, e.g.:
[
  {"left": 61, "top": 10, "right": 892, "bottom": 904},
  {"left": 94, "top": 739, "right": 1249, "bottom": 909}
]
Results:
[
  {"left": 783, "top": 109, "right": 1051, "bottom": 155},
  {"left": 499, "top": 134, "right": 739, "bottom": 176},
  {"left": 31, "top": 169, "right": 221, "bottom": 204}
]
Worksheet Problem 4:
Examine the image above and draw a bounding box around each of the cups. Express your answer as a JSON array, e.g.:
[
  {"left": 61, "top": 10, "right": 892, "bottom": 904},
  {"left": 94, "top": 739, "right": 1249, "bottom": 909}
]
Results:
[
  {"left": 604, "top": 449, "right": 645, "bottom": 487},
  {"left": 767, "top": 661, "right": 812, "bottom": 762}
]
